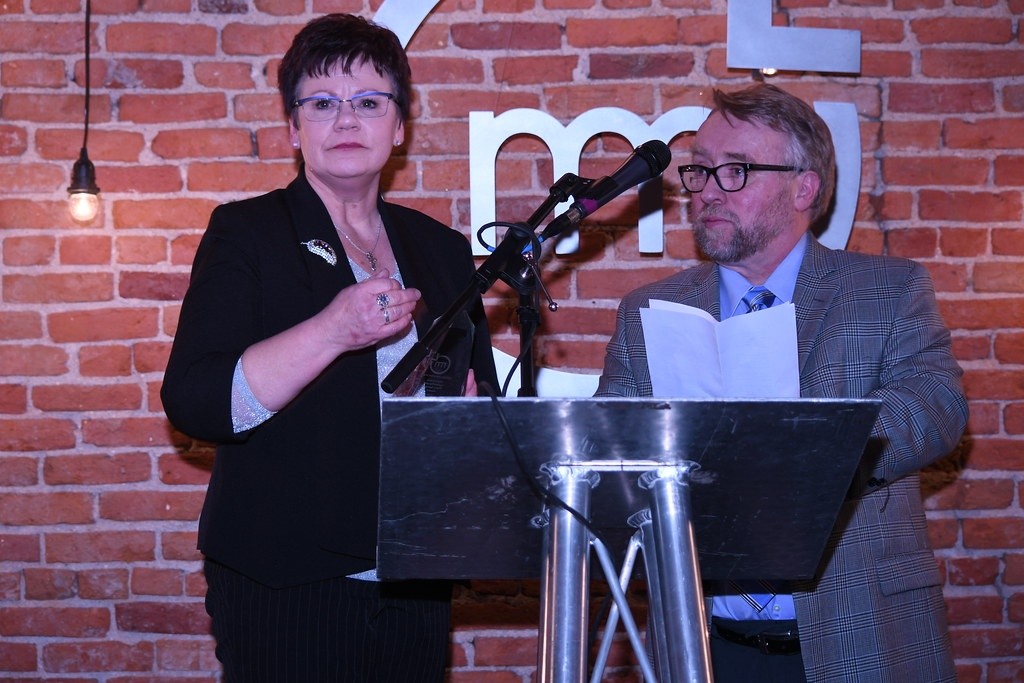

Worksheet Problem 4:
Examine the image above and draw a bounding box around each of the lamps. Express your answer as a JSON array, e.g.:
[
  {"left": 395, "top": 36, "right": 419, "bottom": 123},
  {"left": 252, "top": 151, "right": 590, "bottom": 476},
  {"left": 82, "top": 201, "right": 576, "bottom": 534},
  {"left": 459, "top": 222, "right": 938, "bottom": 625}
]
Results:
[{"left": 65, "top": 1, "right": 104, "bottom": 225}]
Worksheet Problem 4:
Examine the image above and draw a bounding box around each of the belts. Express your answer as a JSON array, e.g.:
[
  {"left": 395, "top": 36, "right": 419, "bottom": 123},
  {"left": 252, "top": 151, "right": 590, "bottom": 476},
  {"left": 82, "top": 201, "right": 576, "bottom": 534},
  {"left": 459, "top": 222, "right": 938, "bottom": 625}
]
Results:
[{"left": 710, "top": 622, "right": 800, "bottom": 656}]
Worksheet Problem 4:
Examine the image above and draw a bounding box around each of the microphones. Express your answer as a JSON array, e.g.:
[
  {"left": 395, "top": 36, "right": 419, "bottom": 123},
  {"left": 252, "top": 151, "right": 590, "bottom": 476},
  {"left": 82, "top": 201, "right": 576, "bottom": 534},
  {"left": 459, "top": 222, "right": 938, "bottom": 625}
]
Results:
[{"left": 540, "top": 139, "right": 671, "bottom": 240}]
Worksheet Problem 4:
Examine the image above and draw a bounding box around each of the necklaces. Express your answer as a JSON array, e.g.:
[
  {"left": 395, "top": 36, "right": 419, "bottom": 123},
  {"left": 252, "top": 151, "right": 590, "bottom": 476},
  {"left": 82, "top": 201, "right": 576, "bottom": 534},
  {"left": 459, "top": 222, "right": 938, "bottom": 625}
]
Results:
[{"left": 334, "top": 213, "right": 382, "bottom": 271}]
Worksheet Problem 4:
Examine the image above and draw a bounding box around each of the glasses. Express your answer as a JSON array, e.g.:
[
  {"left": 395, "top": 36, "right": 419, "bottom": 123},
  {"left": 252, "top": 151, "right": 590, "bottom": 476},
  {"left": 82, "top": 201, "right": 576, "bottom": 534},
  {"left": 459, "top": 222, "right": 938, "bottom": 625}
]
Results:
[
  {"left": 291, "top": 93, "right": 403, "bottom": 117},
  {"left": 677, "top": 161, "right": 803, "bottom": 194}
]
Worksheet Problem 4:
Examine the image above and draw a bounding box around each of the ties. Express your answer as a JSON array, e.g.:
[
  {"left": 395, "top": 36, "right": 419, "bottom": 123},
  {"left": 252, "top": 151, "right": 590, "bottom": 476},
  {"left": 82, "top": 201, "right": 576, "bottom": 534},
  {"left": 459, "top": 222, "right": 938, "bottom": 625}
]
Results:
[{"left": 742, "top": 285, "right": 776, "bottom": 314}]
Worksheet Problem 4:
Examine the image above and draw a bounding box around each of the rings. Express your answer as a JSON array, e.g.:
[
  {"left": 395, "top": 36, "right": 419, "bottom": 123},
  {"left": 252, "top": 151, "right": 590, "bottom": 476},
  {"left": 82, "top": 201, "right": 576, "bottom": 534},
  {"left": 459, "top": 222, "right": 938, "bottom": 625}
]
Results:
[
  {"left": 376, "top": 293, "right": 389, "bottom": 310},
  {"left": 383, "top": 310, "right": 390, "bottom": 325}
]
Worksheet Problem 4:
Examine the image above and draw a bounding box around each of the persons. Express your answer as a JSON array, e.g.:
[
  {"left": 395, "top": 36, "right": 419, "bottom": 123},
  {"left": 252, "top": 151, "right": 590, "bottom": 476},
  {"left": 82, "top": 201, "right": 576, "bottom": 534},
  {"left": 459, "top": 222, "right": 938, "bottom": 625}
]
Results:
[
  {"left": 590, "top": 84, "right": 969, "bottom": 682},
  {"left": 159, "top": 14, "right": 500, "bottom": 681}
]
